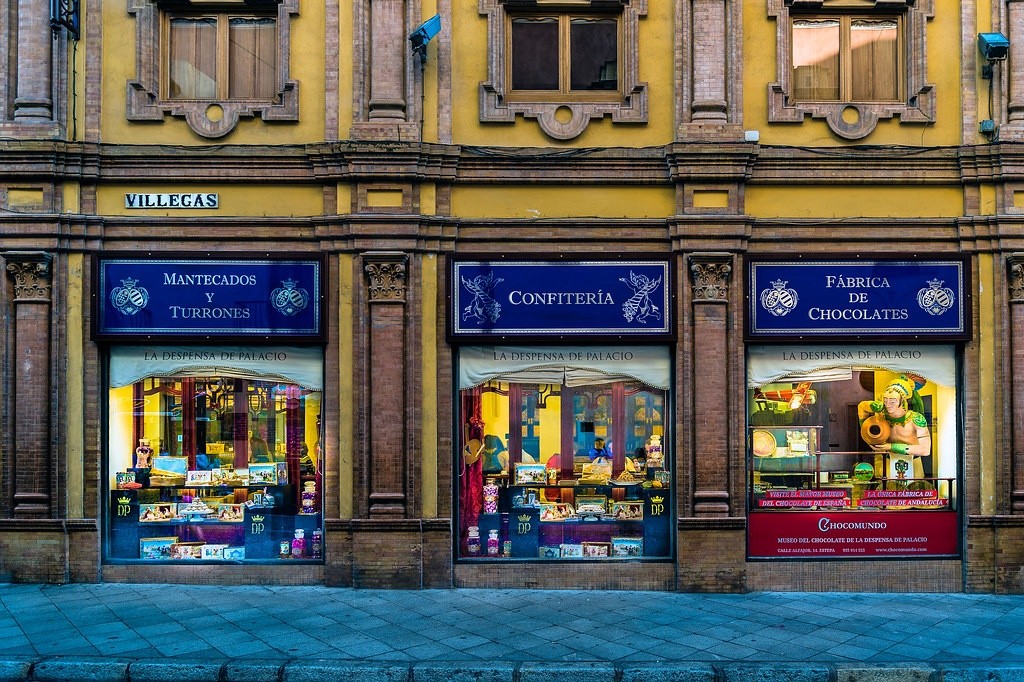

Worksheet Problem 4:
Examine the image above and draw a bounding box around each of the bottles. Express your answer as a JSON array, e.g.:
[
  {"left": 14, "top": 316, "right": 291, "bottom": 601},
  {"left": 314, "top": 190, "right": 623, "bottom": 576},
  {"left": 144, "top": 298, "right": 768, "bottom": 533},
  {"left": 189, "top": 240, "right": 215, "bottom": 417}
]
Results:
[
  {"left": 488, "top": 530, "right": 499, "bottom": 556},
  {"left": 301, "top": 481, "right": 318, "bottom": 514},
  {"left": 291, "top": 529, "right": 307, "bottom": 558},
  {"left": 466, "top": 527, "right": 481, "bottom": 556},
  {"left": 312, "top": 531, "right": 322, "bottom": 559},
  {"left": 482, "top": 478, "right": 498, "bottom": 515}
]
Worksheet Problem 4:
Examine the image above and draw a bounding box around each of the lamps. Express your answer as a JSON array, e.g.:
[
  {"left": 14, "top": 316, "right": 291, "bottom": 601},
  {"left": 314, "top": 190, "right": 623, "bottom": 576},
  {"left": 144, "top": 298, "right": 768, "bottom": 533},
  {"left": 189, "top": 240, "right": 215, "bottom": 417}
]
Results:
[
  {"left": 976, "top": 30, "right": 1011, "bottom": 79},
  {"left": 411, "top": 14, "right": 442, "bottom": 64}
]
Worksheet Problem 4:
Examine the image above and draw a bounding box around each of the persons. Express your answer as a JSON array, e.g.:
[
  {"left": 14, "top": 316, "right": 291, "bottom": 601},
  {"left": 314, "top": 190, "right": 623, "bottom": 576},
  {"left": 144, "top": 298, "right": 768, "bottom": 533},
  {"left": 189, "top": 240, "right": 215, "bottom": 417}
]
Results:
[
  {"left": 250, "top": 437, "right": 272, "bottom": 463},
  {"left": 299, "top": 441, "right": 315, "bottom": 475},
  {"left": 589, "top": 438, "right": 612, "bottom": 462},
  {"left": 543, "top": 452, "right": 561, "bottom": 503},
  {"left": 857, "top": 373, "right": 931, "bottom": 479},
  {"left": 483, "top": 434, "right": 535, "bottom": 471}
]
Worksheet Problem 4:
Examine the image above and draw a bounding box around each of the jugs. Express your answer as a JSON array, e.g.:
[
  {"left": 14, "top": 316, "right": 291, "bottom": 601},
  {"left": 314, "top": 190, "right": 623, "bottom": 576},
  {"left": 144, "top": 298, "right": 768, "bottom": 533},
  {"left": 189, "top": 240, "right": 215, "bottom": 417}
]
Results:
[{"left": 861, "top": 413, "right": 890, "bottom": 446}]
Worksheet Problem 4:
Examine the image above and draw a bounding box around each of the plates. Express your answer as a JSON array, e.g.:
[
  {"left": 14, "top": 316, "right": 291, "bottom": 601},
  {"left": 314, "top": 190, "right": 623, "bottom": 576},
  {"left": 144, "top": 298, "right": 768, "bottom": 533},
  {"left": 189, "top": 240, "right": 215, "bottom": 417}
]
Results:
[{"left": 748, "top": 430, "right": 776, "bottom": 457}]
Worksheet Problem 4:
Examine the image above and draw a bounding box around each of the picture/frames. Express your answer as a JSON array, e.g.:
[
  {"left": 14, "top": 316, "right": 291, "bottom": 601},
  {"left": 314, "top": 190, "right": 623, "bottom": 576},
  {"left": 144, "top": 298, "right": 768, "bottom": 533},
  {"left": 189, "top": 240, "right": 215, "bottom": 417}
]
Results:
[
  {"left": 140, "top": 493, "right": 273, "bottom": 523},
  {"left": 611, "top": 537, "right": 643, "bottom": 557},
  {"left": 139, "top": 537, "right": 245, "bottom": 561},
  {"left": 115, "top": 456, "right": 288, "bottom": 490},
  {"left": 536, "top": 542, "right": 609, "bottom": 559},
  {"left": 510, "top": 462, "right": 645, "bottom": 521}
]
